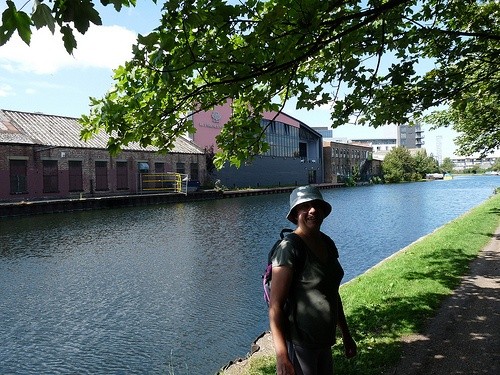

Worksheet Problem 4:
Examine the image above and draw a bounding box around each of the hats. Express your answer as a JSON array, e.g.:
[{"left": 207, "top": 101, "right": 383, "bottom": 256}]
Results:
[{"left": 285, "top": 185, "right": 332, "bottom": 225}]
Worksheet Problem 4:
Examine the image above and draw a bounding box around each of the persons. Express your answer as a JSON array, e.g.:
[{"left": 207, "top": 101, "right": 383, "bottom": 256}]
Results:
[{"left": 268, "top": 185, "right": 361, "bottom": 375}]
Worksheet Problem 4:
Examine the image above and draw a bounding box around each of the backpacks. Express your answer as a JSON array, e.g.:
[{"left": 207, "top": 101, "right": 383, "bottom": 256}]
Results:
[{"left": 260, "top": 228, "right": 307, "bottom": 311}]
